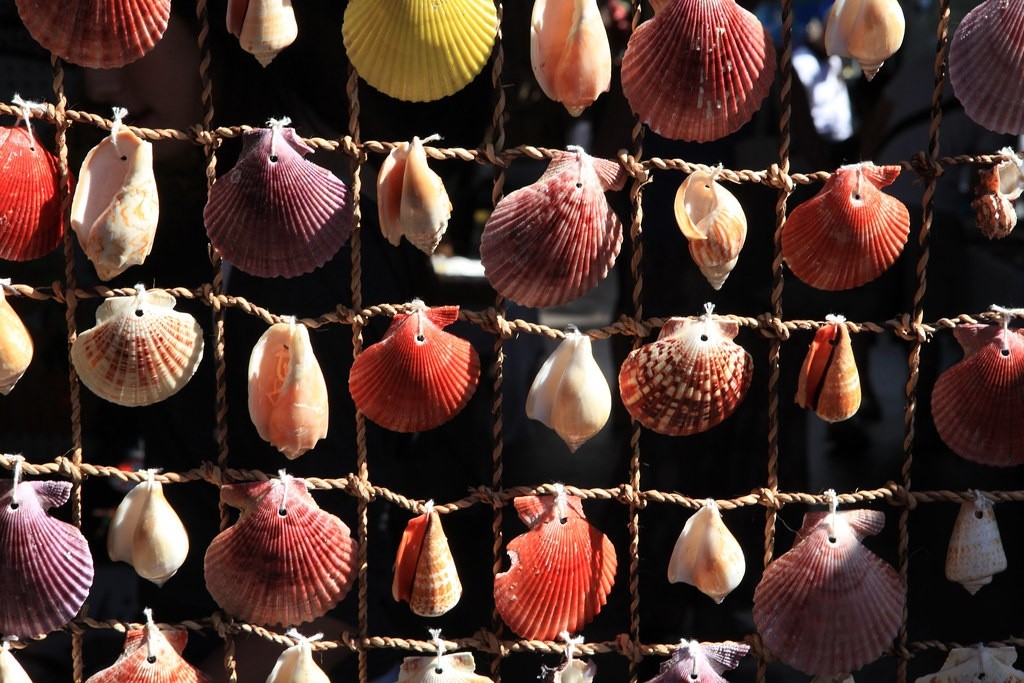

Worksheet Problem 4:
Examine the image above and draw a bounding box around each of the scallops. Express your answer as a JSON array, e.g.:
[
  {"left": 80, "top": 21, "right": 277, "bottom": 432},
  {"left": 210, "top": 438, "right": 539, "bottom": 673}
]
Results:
[{"left": 0, "top": 1, "right": 1024, "bottom": 682}]
